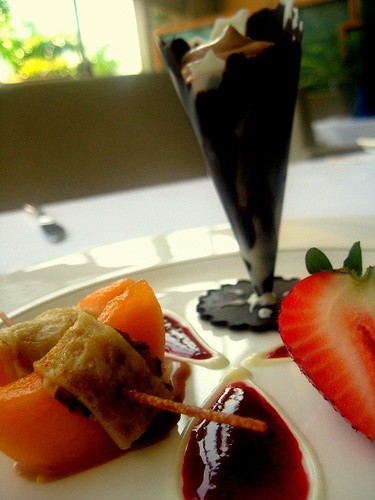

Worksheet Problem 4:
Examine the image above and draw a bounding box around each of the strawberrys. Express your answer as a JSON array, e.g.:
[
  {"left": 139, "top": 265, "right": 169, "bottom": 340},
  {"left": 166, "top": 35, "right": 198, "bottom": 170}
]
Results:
[{"left": 276, "top": 242, "right": 375, "bottom": 442}]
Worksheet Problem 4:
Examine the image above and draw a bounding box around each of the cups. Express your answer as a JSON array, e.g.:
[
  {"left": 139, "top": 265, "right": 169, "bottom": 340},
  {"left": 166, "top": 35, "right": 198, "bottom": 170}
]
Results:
[{"left": 149, "top": 14, "right": 306, "bottom": 330}]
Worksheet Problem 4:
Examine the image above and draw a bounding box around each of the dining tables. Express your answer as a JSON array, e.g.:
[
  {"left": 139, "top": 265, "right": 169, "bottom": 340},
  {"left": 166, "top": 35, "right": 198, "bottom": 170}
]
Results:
[{"left": 0, "top": 150, "right": 375, "bottom": 274}]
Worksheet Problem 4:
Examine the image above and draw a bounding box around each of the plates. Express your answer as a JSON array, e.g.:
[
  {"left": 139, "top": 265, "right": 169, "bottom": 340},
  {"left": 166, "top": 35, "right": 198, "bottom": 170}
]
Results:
[{"left": 0, "top": 244, "right": 375, "bottom": 500}]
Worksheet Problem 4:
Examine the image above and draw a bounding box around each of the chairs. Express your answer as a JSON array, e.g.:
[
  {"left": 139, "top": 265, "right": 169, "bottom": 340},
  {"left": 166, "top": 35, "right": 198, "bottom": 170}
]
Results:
[{"left": 0, "top": 72, "right": 210, "bottom": 213}]
